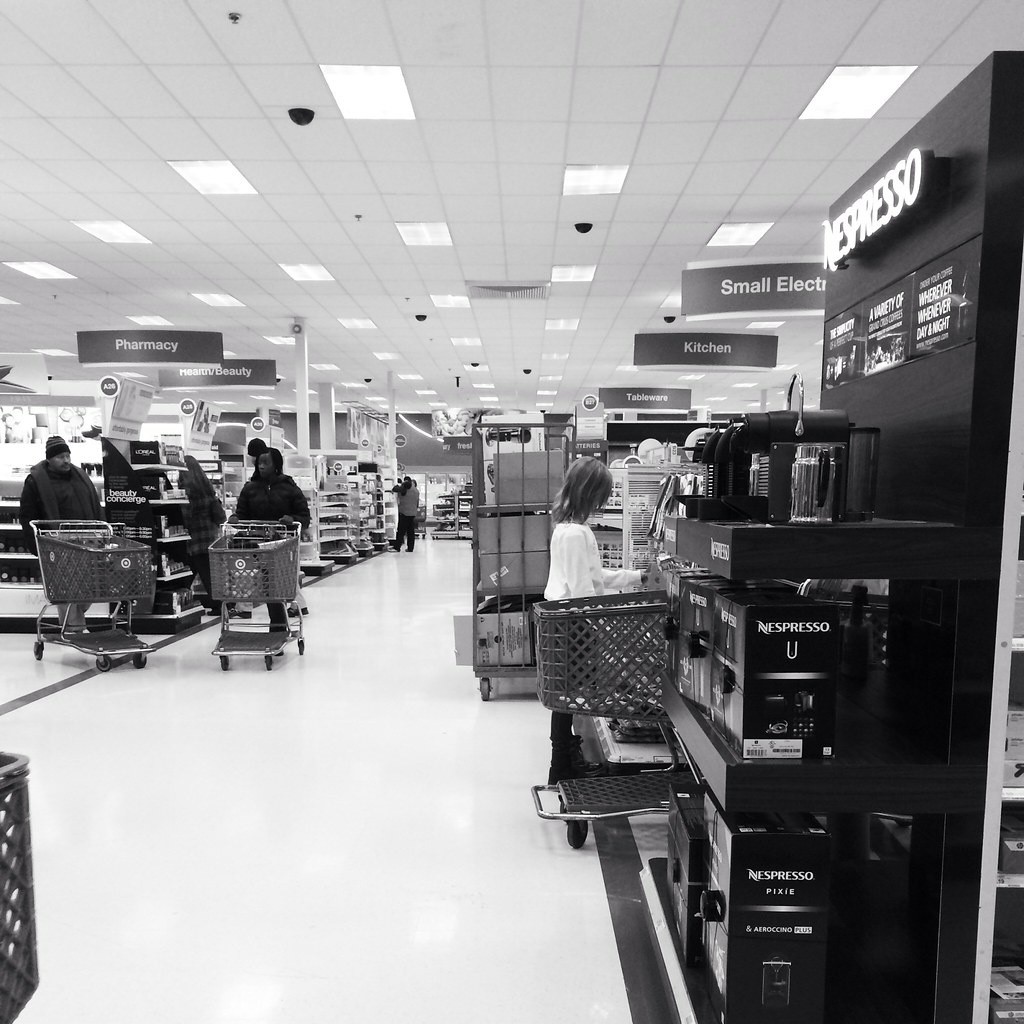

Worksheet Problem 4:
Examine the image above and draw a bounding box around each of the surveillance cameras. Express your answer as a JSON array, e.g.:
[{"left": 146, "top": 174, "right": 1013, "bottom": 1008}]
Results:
[
  {"left": 288, "top": 108, "right": 314, "bottom": 126},
  {"left": 364, "top": 379, "right": 372, "bottom": 382},
  {"left": 48, "top": 376, "right": 53, "bottom": 381},
  {"left": 574, "top": 223, "right": 592, "bottom": 233},
  {"left": 664, "top": 316, "right": 676, "bottom": 323},
  {"left": 540, "top": 410, "right": 546, "bottom": 413},
  {"left": 276, "top": 379, "right": 281, "bottom": 383},
  {"left": 523, "top": 369, "right": 531, "bottom": 374},
  {"left": 471, "top": 363, "right": 479, "bottom": 367}
]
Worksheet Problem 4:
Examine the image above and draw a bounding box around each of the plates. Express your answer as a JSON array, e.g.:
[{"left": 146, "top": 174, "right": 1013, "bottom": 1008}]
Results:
[
  {"left": 685, "top": 428, "right": 712, "bottom": 461},
  {"left": 637, "top": 438, "right": 663, "bottom": 464}
]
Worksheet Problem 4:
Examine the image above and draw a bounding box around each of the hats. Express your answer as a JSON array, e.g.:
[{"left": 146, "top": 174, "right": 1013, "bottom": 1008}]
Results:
[{"left": 46, "top": 436, "right": 71, "bottom": 460}]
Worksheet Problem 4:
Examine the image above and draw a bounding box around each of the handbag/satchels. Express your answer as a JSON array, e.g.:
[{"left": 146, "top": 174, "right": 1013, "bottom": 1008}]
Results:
[{"left": 210, "top": 498, "right": 227, "bottom": 524}]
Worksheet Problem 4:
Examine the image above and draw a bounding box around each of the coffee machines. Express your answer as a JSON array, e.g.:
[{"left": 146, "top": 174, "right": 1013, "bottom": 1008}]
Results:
[{"left": 673, "top": 409, "right": 881, "bottom": 528}]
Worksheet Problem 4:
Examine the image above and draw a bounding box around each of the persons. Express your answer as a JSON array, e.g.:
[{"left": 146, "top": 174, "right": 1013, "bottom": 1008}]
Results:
[
  {"left": 226, "top": 438, "right": 312, "bottom": 632},
  {"left": 543, "top": 456, "right": 652, "bottom": 791},
  {"left": 0, "top": 406, "right": 24, "bottom": 444},
  {"left": 177, "top": 455, "right": 236, "bottom": 617},
  {"left": 18, "top": 436, "right": 108, "bottom": 636},
  {"left": 392, "top": 476, "right": 420, "bottom": 552}
]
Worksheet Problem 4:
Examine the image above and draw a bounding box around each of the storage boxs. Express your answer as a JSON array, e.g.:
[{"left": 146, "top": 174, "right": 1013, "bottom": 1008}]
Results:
[
  {"left": 672, "top": 574, "right": 841, "bottom": 759},
  {"left": 162, "top": 554, "right": 190, "bottom": 576},
  {"left": 321, "top": 529, "right": 348, "bottom": 537},
  {"left": 462, "top": 526, "right": 470, "bottom": 531},
  {"left": 153, "top": 587, "right": 193, "bottom": 615},
  {"left": 452, "top": 603, "right": 531, "bottom": 666},
  {"left": 459, "top": 501, "right": 470, "bottom": 509},
  {"left": 160, "top": 515, "right": 189, "bottom": 538},
  {"left": 138, "top": 477, "right": 186, "bottom": 500},
  {"left": 323, "top": 474, "right": 348, "bottom": 492},
  {"left": 460, "top": 516, "right": 467, "bottom": 520},
  {"left": 665, "top": 782, "right": 832, "bottom": 1024}
]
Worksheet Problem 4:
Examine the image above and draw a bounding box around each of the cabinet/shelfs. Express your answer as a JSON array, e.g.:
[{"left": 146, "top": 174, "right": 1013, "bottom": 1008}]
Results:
[
  {"left": 969, "top": 494, "right": 1024, "bottom": 1024},
  {"left": 316, "top": 455, "right": 359, "bottom": 563},
  {"left": 206, "top": 461, "right": 246, "bottom": 522},
  {"left": 284, "top": 455, "right": 335, "bottom": 577},
  {"left": 458, "top": 495, "right": 474, "bottom": 540},
  {"left": 349, "top": 474, "right": 375, "bottom": 558},
  {"left": 636, "top": 515, "right": 962, "bottom": 1024},
  {"left": 0, "top": 443, "right": 205, "bottom": 633},
  {"left": 471, "top": 424, "right": 577, "bottom": 703},
  {"left": 588, "top": 464, "right": 702, "bottom": 571},
  {"left": 413, "top": 506, "right": 427, "bottom": 539},
  {"left": 430, "top": 496, "right": 459, "bottom": 540},
  {"left": 369, "top": 491, "right": 386, "bottom": 551}
]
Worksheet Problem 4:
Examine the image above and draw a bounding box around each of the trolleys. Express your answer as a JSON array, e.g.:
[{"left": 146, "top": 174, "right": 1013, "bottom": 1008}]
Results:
[
  {"left": 473, "top": 422, "right": 578, "bottom": 701},
  {"left": 531, "top": 590, "right": 701, "bottom": 849},
  {"left": 208, "top": 518, "right": 305, "bottom": 670},
  {"left": 29, "top": 519, "right": 156, "bottom": 672}
]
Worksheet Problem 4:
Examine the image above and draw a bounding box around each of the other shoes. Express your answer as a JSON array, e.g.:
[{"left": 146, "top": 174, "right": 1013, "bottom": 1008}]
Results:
[
  {"left": 389, "top": 547, "right": 400, "bottom": 552},
  {"left": 286, "top": 607, "right": 309, "bottom": 617},
  {"left": 405, "top": 549, "right": 412, "bottom": 552},
  {"left": 207, "top": 609, "right": 221, "bottom": 616},
  {"left": 228, "top": 608, "right": 252, "bottom": 619}
]
